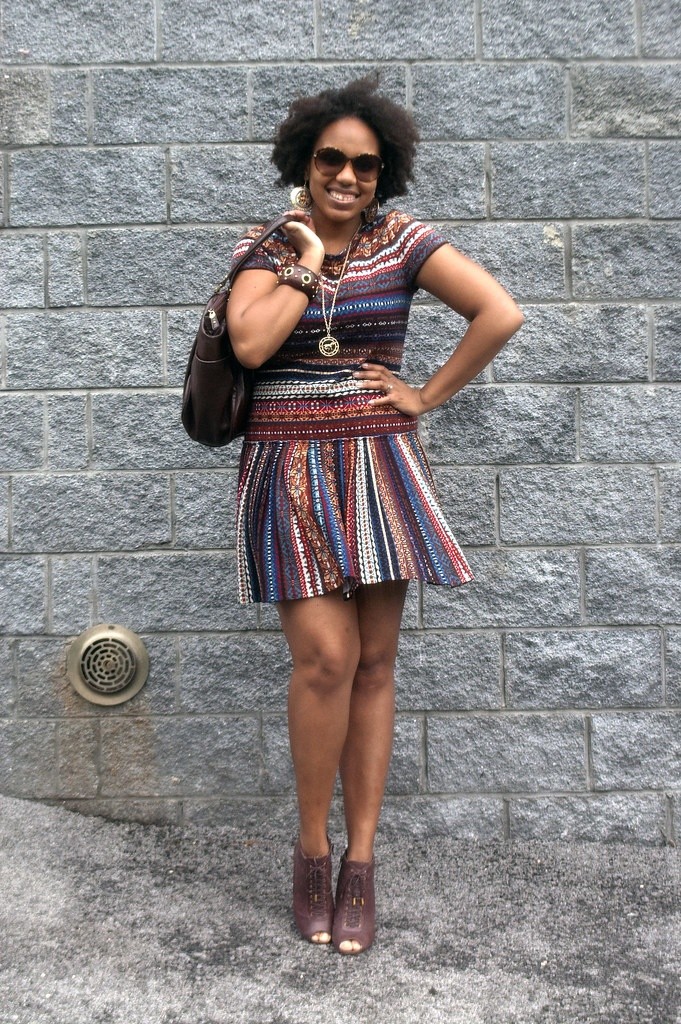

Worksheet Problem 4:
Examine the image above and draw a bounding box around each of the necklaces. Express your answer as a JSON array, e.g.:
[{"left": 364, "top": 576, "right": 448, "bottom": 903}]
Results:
[{"left": 319, "top": 219, "right": 362, "bottom": 357}]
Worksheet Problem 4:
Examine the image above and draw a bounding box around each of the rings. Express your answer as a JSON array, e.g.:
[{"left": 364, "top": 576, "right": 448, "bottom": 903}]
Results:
[{"left": 385, "top": 384, "right": 394, "bottom": 393}]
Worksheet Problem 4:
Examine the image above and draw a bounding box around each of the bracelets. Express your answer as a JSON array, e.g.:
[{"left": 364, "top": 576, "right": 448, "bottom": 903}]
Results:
[{"left": 277, "top": 262, "right": 320, "bottom": 301}]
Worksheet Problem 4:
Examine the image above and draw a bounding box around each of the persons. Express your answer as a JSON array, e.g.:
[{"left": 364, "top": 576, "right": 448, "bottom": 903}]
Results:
[{"left": 226, "top": 88, "right": 523, "bottom": 955}]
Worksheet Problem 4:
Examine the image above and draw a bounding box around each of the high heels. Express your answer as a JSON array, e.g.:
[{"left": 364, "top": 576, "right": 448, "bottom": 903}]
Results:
[
  {"left": 333, "top": 850, "right": 374, "bottom": 955},
  {"left": 292, "top": 834, "right": 334, "bottom": 944}
]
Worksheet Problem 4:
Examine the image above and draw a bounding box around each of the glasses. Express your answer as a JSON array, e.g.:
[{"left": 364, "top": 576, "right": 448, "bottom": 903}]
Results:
[{"left": 310, "top": 147, "right": 386, "bottom": 183}]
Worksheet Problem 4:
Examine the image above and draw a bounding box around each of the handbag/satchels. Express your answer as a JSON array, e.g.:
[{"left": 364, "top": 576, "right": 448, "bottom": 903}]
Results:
[{"left": 181, "top": 215, "right": 299, "bottom": 446}]
